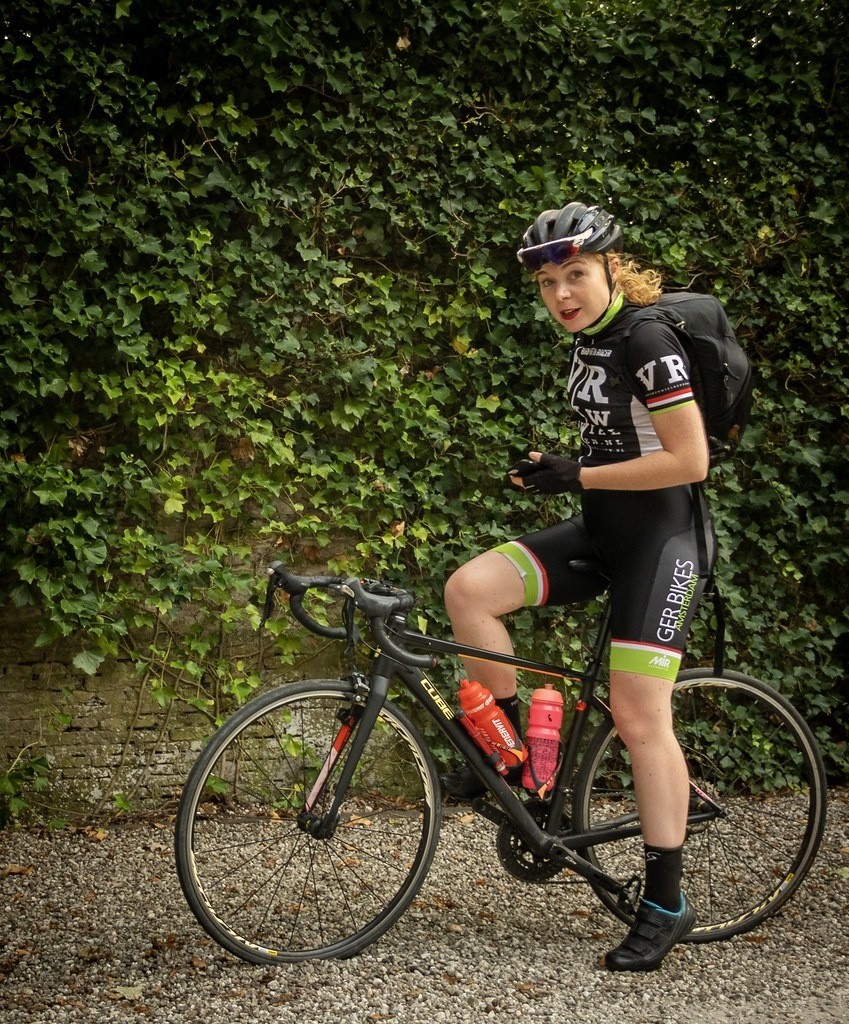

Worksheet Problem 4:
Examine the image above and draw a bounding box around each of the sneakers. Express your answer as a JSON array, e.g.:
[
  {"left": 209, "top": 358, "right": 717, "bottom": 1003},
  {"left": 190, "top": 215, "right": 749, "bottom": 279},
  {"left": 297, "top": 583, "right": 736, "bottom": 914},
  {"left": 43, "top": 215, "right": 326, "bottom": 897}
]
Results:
[
  {"left": 605, "top": 891, "right": 697, "bottom": 973},
  {"left": 439, "top": 762, "right": 524, "bottom": 802}
]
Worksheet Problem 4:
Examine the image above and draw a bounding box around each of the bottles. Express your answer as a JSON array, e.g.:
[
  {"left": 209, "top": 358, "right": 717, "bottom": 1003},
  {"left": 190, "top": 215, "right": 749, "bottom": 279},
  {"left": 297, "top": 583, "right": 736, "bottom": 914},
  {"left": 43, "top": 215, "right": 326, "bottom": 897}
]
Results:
[
  {"left": 522, "top": 684, "right": 564, "bottom": 792},
  {"left": 457, "top": 679, "right": 530, "bottom": 768}
]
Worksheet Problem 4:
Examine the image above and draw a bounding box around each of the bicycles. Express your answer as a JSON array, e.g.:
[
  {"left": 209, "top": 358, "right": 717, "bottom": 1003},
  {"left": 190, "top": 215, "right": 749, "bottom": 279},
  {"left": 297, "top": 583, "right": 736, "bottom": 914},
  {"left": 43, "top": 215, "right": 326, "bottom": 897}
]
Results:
[{"left": 171, "top": 560, "right": 832, "bottom": 966}]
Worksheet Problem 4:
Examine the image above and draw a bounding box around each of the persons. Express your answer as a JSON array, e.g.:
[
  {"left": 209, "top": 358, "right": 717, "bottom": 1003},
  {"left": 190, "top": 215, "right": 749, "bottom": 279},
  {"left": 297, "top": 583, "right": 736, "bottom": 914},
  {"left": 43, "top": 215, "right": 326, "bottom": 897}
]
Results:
[{"left": 443, "top": 202, "right": 720, "bottom": 971}]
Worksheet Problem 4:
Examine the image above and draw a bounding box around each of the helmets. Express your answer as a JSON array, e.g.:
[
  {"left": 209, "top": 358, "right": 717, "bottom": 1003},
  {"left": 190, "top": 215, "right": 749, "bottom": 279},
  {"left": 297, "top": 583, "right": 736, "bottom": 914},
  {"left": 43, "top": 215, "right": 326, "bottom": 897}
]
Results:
[{"left": 517, "top": 201, "right": 623, "bottom": 275}]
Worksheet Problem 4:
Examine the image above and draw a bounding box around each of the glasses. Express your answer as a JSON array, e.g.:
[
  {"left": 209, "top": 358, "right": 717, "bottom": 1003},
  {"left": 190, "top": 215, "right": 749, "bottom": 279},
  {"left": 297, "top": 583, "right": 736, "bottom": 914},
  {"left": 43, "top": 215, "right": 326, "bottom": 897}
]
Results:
[{"left": 517, "top": 228, "right": 593, "bottom": 273}]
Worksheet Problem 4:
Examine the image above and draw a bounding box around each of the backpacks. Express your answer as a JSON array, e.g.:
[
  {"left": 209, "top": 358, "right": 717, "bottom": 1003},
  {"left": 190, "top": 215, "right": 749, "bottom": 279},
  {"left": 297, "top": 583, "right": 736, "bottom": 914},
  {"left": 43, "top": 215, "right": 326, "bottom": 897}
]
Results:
[{"left": 619, "top": 292, "right": 751, "bottom": 470}]
